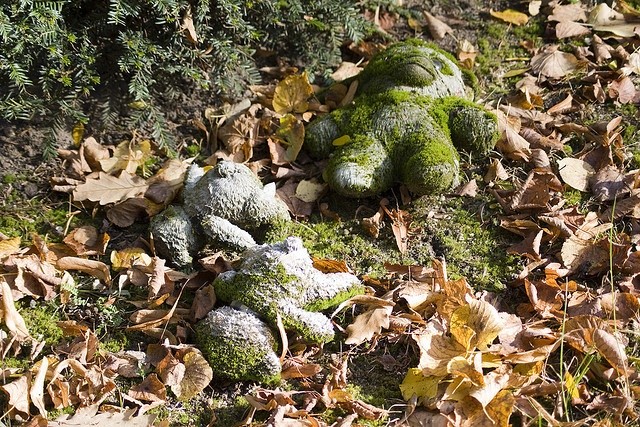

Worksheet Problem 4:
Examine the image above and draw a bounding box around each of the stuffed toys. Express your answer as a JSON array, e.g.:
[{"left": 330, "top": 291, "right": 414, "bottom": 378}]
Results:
[
  {"left": 147, "top": 160, "right": 292, "bottom": 269},
  {"left": 304, "top": 37, "right": 501, "bottom": 200},
  {"left": 195, "top": 234, "right": 365, "bottom": 388}
]
